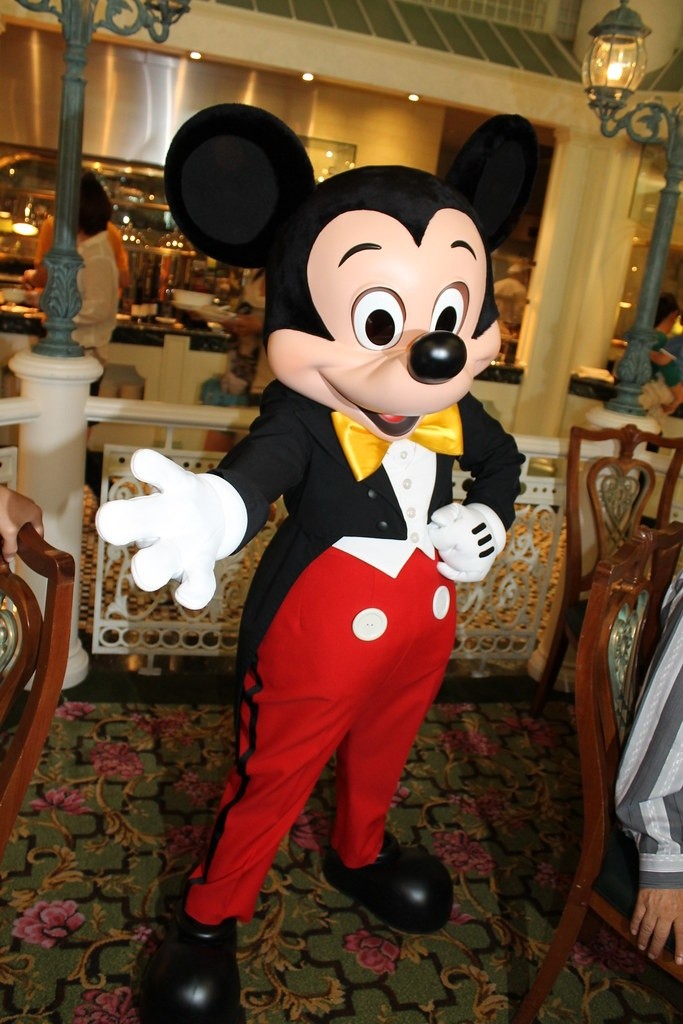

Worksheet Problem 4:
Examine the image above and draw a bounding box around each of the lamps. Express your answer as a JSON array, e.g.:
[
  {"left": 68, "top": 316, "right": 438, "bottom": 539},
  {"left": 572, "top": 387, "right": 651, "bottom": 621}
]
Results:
[
  {"left": 13, "top": 196, "right": 39, "bottom": 237},
  {"left": 580, "top": 0, "right": 683, "bottom": 417},
  {"left": 119, "top": 223, "right": 146, "bottom": 310},
  {"left": 154, "top": 226, "right": 197, "bottom": 318}
]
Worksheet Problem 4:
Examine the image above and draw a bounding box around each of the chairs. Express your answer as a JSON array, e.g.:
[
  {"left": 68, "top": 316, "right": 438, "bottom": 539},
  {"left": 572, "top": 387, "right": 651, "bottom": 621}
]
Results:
[
  {"left": 531, "top": 424, "right": 683, "bottom": 718},
  {"left": 511, "top": 521, "right": 683, "bottom": 1024},
  {"left": 0, "top": 524, "right": 74, "bottom": 857}
]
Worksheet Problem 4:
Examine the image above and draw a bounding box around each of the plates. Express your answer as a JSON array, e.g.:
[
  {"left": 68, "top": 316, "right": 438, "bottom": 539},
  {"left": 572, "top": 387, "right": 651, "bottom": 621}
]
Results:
[
  {"left": 156, "top": 316, "right": 176, "bottom": 324},
  {"left": 172, "top": 302, "right": 236, "bottom": 320},
  {"left": 1, "top": 306, "right": 36, "bottom": 314}
]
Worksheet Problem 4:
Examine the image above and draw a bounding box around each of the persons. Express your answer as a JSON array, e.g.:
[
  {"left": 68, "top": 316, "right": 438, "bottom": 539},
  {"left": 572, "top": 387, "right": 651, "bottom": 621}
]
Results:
[
  {"left": 612, "top": 293, "right": 683, "bottom": 418},
  {"left": 21, "top": 171, "right": 132, "bottom": 349},
  {"left": 0, "top": 485, "right": 45, "bottom": 564},
  {"left": 614, "top": 575, "right": 683, "bottom": 970},
  {"left": 186, "top": 267, "right": 284, "bottom": 531}
]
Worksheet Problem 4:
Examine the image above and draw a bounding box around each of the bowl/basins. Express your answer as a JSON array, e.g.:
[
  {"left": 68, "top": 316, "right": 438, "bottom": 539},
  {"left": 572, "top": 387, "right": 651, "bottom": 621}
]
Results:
[
  {"left": 0, "top": 288, "right": 27, "bottom": 304},
  {"left": 170, "top": 289, "right": 216, "bottom": 304}
]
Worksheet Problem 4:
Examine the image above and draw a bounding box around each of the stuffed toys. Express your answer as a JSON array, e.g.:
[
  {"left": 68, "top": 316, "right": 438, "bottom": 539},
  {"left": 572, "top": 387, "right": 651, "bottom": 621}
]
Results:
[{"left": 91, "top": 100, "right": 539, "bottom": 1024}]
